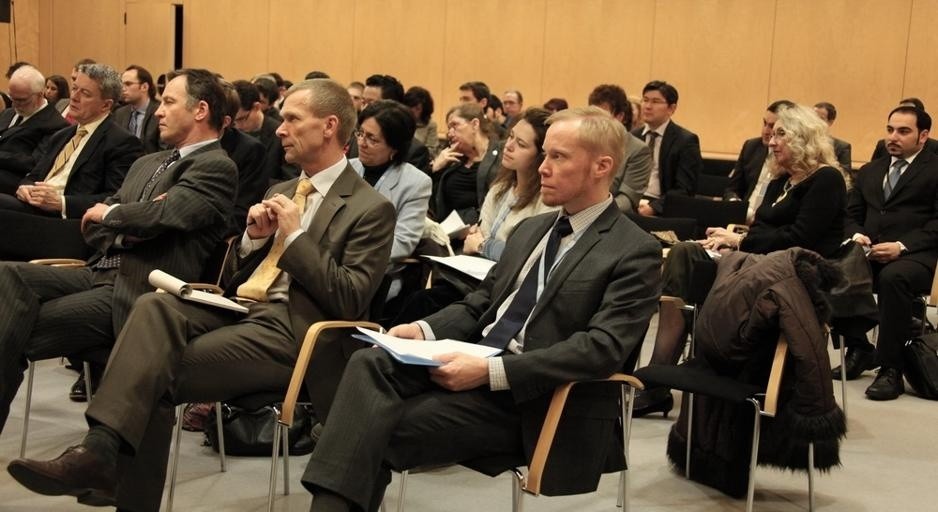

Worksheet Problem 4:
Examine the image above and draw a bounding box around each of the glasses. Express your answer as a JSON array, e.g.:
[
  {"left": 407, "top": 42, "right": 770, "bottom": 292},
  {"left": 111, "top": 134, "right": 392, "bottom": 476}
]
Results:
[{"left": 353, "top": 130, "right": 380, "bottom": 147}]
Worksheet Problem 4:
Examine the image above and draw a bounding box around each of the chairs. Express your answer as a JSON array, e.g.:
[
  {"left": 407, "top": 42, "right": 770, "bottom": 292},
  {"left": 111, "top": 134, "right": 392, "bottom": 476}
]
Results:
[{"left": 0, "top": 214, "right": 938, "bottom": 512}]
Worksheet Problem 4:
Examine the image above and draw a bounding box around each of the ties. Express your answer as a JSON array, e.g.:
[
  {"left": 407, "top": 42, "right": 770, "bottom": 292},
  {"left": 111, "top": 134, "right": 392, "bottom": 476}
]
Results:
[
  {"left": 647, "top": 130, "right": 659, "bottom": 161},
  {"left": 475, "top": 216, "right": 574, "bottom": 351},
  {"left": 128, "top": 110, "right": 141, "bottom": 137},
  {"left": 44, "top": 126, "right": 88, "bottom": 183},
  {"left": 882, "top": 159, "right": 909, "bottom": 202},
  {"left": 235, "top": 179, "right": 316, "bottom": 304},
  {"left": 96, "top": 150, "right": 181, "bottom": 269},
  {"left": 13, "top": 115, "right": 24, "bottom": 127}
]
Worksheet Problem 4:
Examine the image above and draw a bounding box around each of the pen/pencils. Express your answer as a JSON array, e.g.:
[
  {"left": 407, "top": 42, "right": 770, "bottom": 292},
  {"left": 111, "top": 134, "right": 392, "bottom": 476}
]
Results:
[
  {"left": 477, "top": 219, "right": 482, "bottom": 227},
  {"left": 246, "top": 221, "right": 256, "bottom": 226},
  {"left": 448, "top": 141, "right": 452, "bottom": 149}
]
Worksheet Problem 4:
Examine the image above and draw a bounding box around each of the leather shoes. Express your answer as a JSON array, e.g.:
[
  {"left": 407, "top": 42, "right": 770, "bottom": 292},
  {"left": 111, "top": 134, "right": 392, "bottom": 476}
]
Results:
[
  {"left": 5, "top": 443, "right": 120, "bottom": 508},
  {"left": 865, "top": 367, "right": 906, "bottom": 401},
  {"left": 831, "top": 344, "right": 881, "bottom": 382},
  {"left": 69, "top": 369, "right": 99, "bottom": 402}
]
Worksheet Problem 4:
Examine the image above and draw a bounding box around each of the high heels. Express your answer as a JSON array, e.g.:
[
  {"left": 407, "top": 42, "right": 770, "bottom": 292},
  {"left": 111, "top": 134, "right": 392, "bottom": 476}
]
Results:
[{"left": 616, "top": 394, "right": 675, "bottom": 419}]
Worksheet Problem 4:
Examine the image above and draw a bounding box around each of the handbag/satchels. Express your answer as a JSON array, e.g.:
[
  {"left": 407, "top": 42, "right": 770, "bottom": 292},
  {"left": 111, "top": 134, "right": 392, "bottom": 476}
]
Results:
[
  {"left": 902, "top": 332, "right": 938, "bottom": 402},
  {"left": 204, "top": 402, "right": 318, "bottom": 457}
]
